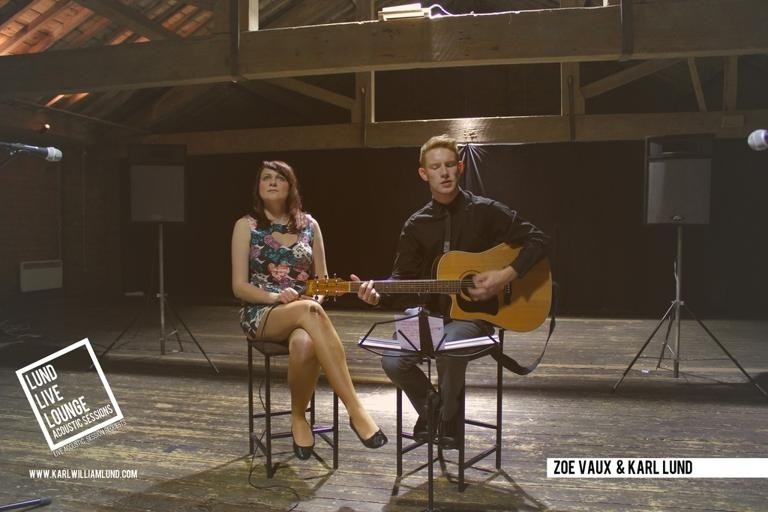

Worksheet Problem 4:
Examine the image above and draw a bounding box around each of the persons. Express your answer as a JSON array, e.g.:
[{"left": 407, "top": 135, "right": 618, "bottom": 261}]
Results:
[
  {"left": 230, "top": 160, "right": 389, "bottom": 462},
  {"left": 348, "top": 134, "right": 554, "bottom": 449}
]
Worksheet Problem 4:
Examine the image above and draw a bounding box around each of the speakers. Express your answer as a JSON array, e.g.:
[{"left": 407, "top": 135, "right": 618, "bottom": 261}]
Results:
[
  {"left": 644, "top": 134, "right": 714, "bottom": 227},
  {"left": 128, "top": 144, "right": 186, "bottom": 223}
]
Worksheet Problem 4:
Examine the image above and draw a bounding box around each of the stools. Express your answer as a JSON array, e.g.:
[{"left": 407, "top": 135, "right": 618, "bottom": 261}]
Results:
[{"left": 246, "top": 337, "right": 338, "bottom": 479}]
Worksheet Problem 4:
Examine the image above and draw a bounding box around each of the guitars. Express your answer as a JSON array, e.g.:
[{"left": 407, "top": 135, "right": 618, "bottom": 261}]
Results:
[{"left": 305, "top": 242, "right": 552, "bottom": 332}]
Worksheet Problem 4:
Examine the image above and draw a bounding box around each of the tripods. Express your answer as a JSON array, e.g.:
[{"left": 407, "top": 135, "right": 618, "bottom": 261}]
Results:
[
  {"left": 613, "top": 225, "right": 767, "bottom": 398},
  {"left": 90, "top": 223, "right": 219, "bottom": 375}
]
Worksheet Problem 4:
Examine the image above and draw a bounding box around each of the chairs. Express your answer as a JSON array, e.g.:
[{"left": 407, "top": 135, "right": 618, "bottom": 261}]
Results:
[{"left": 397, "top": 329, "right": 504, "bottom": 491}]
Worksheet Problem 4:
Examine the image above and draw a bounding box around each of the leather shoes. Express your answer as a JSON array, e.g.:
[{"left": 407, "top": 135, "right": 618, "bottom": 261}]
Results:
[
  {"left": 350, "top": 416, "right": 387, "bottom": 448},
  {"left": 291, "top": 424, "right": 315, "bottom": 460},
  {"left": 413, "top": 415, "right": 456, "bottom": 450}
]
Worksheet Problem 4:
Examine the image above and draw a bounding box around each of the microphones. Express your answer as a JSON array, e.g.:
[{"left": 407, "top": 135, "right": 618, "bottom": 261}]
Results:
[
  {"left": 0, "top": 142, "right": 63, "bottom": 162},
  {"left": 298, "top": 287, "right": 307, "bottom": 296},
  {"left": 748, "top": 129, "right": 768, "bottom": 151}
]
